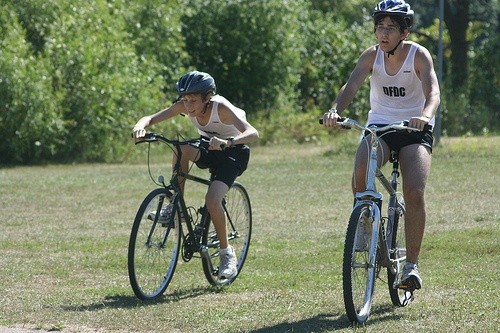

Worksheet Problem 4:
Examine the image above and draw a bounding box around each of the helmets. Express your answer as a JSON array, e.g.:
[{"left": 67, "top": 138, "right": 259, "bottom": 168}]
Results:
[
  {"left": 373, "top": 0, "right": 414, "bottom": 18},
  {"left": 175, "top": 71, "right": 217, "bottom": 95}
]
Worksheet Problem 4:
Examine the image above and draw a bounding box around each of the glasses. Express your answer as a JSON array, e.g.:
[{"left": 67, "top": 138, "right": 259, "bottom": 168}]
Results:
[{"left": 374, "top": 23, "right": 399, "bottom": 33}]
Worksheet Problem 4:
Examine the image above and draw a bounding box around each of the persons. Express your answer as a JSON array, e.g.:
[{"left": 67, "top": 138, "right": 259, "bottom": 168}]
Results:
[
  {"left": 323, "top": 0, "right": 440, "bottom": 289},
  {"left": 132, "top": 71, "right": 260, "bottom": 280}
]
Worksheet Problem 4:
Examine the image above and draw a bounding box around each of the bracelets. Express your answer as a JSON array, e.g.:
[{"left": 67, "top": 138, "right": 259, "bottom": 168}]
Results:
[
  {"left": 226, "top": 136, "right": 234, "bottom": 148},
  {"left": 328, "top": 108, "right": 340, "bottom": 116}
]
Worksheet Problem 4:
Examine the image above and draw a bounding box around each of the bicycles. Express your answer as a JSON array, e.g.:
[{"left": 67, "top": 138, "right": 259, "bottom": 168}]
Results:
[
  {"left": 318, "top": 115, "right": 433, "bottom": 323},
  {"left": 127, "top": 131, "right": 253, "bottom": 300}
]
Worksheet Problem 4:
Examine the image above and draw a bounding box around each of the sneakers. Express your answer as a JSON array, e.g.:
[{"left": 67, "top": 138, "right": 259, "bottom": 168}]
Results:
[
  {"left": 218, "top": 246, "right": 238, "bottom": 279},
  {"left": 147, "top": 207, "right": 174, "bottom": 223},
  {"left": 400, "top": 263, "right": 423, "bottom": 290},
  {"left": 355, "top": 221, "right": 370, "bottom": 252}
]
